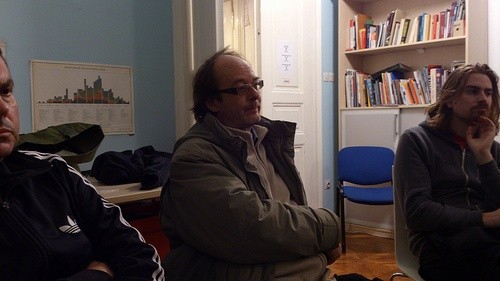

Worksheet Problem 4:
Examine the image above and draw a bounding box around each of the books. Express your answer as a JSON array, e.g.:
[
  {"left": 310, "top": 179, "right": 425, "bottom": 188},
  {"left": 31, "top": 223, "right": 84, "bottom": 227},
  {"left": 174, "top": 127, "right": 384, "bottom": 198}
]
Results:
[{"left": 345, "top": 1, "right": 466, "bottom": 107}]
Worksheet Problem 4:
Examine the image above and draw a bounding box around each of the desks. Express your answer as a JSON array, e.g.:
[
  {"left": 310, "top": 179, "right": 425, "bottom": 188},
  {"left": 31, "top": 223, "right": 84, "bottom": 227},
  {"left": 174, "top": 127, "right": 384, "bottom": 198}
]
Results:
[{"left": 86, "top": 176, "right": 162, "bottom": 204}]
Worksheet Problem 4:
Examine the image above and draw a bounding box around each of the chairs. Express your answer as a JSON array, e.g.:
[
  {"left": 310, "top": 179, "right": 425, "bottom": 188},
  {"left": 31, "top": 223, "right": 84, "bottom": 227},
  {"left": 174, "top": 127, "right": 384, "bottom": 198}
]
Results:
[
  {"left": 389, "top": 163, "right": 425, "bottom": 281},
  {"left": 335, "top": 146, "right": 395, "bottom": 254}
]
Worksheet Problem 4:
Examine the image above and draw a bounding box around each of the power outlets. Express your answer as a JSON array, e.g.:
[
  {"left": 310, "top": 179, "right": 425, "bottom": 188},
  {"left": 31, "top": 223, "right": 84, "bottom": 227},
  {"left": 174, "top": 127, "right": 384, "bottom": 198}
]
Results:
[{"left": 324, "top": 180, "right": 331, "bottom": 190}]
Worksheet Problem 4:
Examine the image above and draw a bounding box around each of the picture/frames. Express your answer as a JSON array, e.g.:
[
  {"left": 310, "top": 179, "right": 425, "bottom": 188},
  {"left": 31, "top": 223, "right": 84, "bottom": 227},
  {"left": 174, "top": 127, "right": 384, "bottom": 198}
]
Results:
[{"left": 30, "top": 59, "right": 135, "bottom": 136}]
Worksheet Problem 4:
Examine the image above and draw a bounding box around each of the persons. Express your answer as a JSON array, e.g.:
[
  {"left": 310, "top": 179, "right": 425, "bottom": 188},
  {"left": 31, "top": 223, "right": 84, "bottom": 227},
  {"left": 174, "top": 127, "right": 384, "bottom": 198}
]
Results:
[
  {"left": 0, "top": 50, "right": 164, "bottom": 281},
  {"left": 393, "top": 63, "right": 500, "bottom": 281},
  {"left": 159, "top": 46, "right": 383, "bottom": 281}
]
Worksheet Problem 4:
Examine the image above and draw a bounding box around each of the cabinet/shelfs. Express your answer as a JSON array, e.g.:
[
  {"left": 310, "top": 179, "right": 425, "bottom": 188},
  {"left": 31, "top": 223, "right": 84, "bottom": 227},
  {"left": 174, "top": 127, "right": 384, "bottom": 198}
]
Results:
[{"left": 338, "top": 0, "right": 488, "bottom": 241}]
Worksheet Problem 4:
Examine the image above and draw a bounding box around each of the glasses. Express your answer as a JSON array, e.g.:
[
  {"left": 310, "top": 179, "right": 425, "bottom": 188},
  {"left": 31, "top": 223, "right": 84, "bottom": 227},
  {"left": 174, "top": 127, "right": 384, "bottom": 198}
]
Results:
[{"left": 215, "top": 80, "right": 263, "bottom": 99}]
